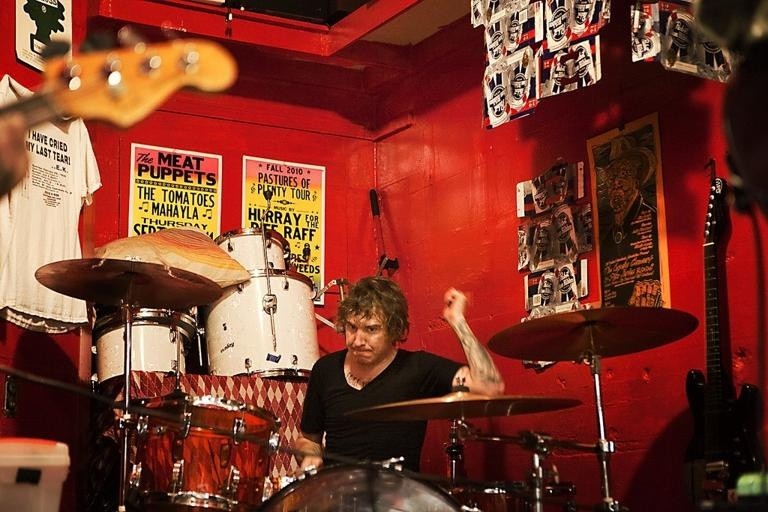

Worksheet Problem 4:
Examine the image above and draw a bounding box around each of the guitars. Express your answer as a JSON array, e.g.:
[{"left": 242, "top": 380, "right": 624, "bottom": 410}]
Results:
[
  {"left": 685, "top": 178, "right": 766, "bottom": 510},
  {"left": 0, "top": 22, "right": 238, "bottom": 129}
]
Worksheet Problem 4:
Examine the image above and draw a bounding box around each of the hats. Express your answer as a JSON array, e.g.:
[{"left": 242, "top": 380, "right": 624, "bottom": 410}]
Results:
[{"left": 595, "top": 136, "right": 658, "bottom": 188}]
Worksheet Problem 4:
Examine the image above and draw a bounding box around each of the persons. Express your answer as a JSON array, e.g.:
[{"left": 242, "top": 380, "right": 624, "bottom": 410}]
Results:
[{"left": 292, "top": 275, "right": 506, "bottom": 478}]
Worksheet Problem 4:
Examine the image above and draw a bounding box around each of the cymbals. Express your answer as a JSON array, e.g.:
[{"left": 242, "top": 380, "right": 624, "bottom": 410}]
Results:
[
  {"left": 342, "top": 391, "right": 582, "bottom": 421},
  {"left": 487, "top": 307, "right": 699, "bottom": 360},
  {"left": 35, "top": 258, "right": 224, "bottom": 309}
]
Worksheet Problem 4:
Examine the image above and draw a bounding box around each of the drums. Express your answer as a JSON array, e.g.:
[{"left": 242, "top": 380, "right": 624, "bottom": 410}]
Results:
[
  {"left": 215, "top": 229, "right": 290, "bottom": 272},
  {"left": 129, "top": 400, "right": 181, "bottom": 506},
  {"left": 198, "top": 269, "right": 320, "bottom": 380},
  {"left": 140, "top": 395, "right": 284, "bottom": 511},
  {"left": 260, "top": 467, "right": 469, "bottom": 511},
  {"left": 93, "top": 312, "right": 187, "bottom": 382}
]
